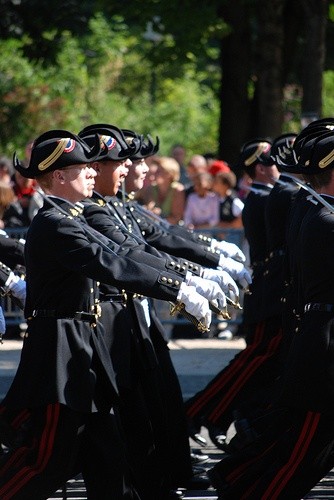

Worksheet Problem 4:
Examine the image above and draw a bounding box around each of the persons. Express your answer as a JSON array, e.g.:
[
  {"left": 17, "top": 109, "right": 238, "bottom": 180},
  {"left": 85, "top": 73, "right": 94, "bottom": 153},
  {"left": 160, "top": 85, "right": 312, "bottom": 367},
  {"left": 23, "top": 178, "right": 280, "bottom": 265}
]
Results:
[{"left": 0, "top": 117, "right": 334, "bottom": 500}]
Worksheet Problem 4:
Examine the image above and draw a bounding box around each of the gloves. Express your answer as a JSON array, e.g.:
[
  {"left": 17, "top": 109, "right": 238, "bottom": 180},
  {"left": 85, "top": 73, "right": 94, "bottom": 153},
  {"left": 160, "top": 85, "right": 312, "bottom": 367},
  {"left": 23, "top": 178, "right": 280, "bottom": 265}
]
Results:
[
  {"left": 186, "top": 270, "right": 227, "bottom": 308},
  {"left": 219, "top": 254, "right": 252, "bottom": 284},
  {"left": 177, "top": 282, "right": 212, "bottom": 329},
  {"left": 202, "top": 267, "right": 240, "bottom": 300},
  {"left": 211, "top": 239, "right": 246, "bottom": 262},
  {"left": 5, "top": 272, "right": 26, "bottom": 306}
]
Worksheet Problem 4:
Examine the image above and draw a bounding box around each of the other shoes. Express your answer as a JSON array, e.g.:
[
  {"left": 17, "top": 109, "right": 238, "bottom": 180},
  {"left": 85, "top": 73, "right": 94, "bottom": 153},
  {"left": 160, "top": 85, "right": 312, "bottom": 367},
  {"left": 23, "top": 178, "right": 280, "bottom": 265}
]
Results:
[{"left": 218, "top": 329, "right": 232, "bottom": 339}]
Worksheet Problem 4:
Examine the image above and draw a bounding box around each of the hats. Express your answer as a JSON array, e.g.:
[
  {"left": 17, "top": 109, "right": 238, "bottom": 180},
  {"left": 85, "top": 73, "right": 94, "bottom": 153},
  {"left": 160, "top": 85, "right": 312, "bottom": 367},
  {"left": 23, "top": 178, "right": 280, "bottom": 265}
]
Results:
[
  {"left": 208, "top": 160, "right": 231, "bottom": 176},
  {"left": 78, "top": 124, "right": 142, "bottom": 161},
  {"left": 293, "top": 118, "right": 334, "bottom": 175},
  {"left": 271, "top": 133, "right": 304, "bottom": 174},
  {"left": 240, "top": 139, "right": 272, "bottom": 171},
  {"left": 121, "top": 128, "right": 160, "bottom": 159},
  {"left": 12, "top": 130, "right": 110, "bottom": 179}
]
